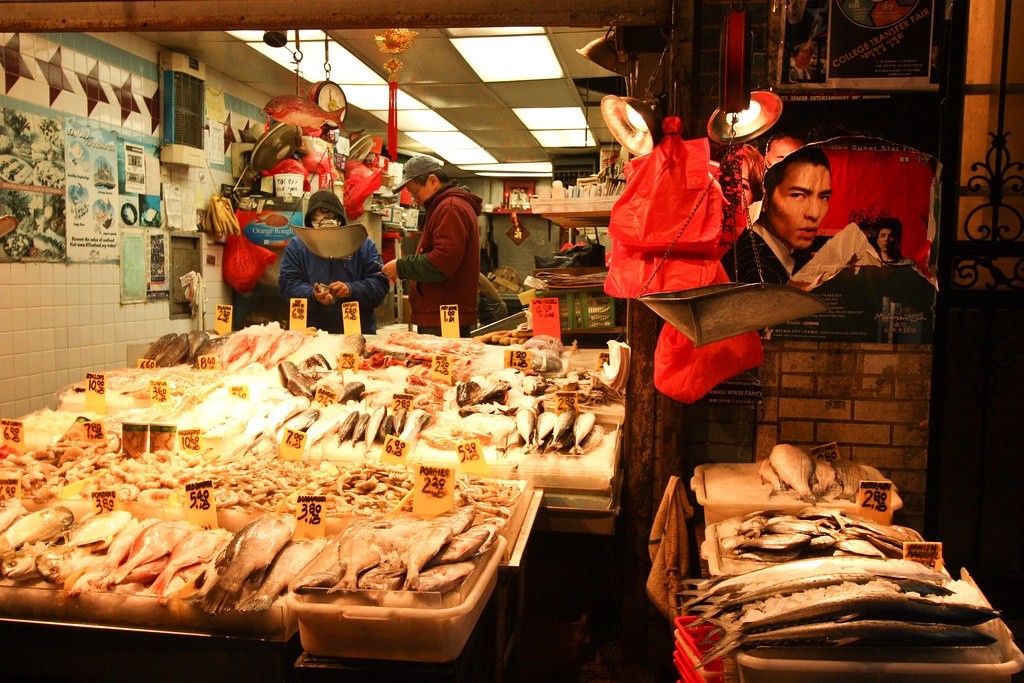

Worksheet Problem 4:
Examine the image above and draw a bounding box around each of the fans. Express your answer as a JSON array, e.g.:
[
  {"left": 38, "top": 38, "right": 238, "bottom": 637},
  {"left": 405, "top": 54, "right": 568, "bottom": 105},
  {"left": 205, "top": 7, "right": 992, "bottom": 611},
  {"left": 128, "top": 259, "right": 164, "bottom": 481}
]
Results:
[
  {"left": 250, "top": 121, "right": 303, "bottom": 175},
  {"left": 342, "top": 130, "right": 374, "bottom": 169}
]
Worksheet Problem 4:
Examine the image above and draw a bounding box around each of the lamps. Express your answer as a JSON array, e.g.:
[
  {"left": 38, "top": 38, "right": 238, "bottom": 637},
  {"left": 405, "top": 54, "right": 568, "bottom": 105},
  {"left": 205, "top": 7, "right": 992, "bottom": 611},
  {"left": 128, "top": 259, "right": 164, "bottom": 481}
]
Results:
[
  {"left": 706, "top": 87, "right": 783, "bottom": 144},
  {"left": 598, "top": 92, "right": 672, "bottom": 156},
  {"left": 575, "top": 28, "right": 636, "bottom": 79}
]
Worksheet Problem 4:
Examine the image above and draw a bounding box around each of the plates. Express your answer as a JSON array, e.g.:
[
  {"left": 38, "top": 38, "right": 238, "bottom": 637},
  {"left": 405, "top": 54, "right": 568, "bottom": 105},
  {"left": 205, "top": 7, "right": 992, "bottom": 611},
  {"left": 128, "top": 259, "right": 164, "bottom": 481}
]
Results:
[
  {"left": 121, "top": 202, "right": 138, "bottom": 225},
  {"left": 0, "top": 154, "right": 33, "bottom": 184}
]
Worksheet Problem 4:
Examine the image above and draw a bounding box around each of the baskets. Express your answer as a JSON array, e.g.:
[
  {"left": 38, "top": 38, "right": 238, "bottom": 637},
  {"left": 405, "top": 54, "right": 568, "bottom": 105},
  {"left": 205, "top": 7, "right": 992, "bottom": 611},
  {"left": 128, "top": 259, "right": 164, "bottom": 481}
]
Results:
[{"left": 673, "top": 615, "right": 723, "bottom": 683}]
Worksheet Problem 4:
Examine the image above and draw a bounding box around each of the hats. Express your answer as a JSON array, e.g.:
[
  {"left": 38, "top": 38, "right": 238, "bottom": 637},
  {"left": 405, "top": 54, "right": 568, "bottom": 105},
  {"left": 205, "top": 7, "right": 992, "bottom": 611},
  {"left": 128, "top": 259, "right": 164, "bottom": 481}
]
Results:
[{"left": 391, "top": 155, "right": 441, "bottom": 195}]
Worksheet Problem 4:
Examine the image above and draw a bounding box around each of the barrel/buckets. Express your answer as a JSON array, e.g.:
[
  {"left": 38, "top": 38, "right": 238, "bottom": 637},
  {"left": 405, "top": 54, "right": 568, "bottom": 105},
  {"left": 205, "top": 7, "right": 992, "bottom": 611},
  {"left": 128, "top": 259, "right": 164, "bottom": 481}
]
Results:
[{"left": 274, "top": 173, "right": 304, "bottom": 198}]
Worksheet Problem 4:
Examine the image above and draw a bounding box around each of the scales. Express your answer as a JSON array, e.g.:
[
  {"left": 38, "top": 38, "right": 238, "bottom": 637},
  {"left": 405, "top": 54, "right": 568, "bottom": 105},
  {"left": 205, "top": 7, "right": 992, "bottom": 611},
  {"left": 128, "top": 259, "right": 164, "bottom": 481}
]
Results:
[
  {"left": 634, "top": 11, "right": 831, "bottom": 348},
  {"left": 287, "top": 73, "right": 369, "bottom": 259}
]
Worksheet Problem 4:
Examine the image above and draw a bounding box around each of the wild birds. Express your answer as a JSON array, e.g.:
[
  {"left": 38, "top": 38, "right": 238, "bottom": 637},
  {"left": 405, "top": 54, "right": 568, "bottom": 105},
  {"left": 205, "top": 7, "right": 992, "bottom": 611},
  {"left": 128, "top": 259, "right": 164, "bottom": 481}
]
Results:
[{"left": 760, "top": 443, "right": 870, "bottom": 503}]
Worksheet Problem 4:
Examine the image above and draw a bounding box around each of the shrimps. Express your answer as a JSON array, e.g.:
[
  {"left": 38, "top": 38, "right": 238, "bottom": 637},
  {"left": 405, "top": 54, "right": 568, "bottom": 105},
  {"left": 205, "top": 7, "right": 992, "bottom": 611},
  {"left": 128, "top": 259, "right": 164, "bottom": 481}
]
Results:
[
  {"left": 83, "top": 446, "right": 223, "bottom": 505},
  {"left": 454, "top": 475, "right": 520, "bottom": 516}
]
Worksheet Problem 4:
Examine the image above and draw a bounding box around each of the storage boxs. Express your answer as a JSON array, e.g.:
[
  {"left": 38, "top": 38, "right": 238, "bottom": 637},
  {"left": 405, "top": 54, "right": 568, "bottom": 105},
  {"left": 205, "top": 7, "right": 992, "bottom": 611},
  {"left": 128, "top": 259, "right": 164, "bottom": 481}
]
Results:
[
  {"left": 536, "top": 285, "right": 618, "bottom": 332},
  {"left": 288, "top": 533, "right": 510, "bottom": 664},
  {"left": 689, "top": 460, "right": 1024, "bottom": 683}
]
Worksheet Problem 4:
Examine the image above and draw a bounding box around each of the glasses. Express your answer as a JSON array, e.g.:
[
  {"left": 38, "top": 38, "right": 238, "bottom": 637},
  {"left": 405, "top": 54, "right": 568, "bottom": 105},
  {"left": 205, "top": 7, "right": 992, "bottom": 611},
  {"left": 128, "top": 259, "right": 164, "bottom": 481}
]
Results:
[{"left": 411, "top": 186, "right": 422, "bottom": 200}]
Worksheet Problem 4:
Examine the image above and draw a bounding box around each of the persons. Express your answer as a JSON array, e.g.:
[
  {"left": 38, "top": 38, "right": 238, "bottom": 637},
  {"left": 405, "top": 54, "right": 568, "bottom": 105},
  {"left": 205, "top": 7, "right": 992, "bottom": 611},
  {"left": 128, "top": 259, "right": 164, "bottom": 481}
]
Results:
[
  {"left": 718, "top": 134, "right": 919, "bottom": 284},
  {"left": 381, "top": 153, "right": 484, "bottom": 337},
  {"left": 788, "top": 44, "right": 815, "bottom": 83},
  {"left": 278, "top": 190, "right": 390, "bottom": 334}
]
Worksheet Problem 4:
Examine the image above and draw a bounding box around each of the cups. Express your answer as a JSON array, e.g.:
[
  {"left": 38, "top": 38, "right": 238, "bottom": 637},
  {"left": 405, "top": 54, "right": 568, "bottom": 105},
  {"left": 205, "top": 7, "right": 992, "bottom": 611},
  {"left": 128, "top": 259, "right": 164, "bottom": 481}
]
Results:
[
  {"left": 122, "top": 423, "right": 149, "bottom": 459},
  {"left": 485, "top": 205, "right": 493, "bottom": 212},
  {"left": 149, "top": 424, "right": 177, "bottom": 453},
  {"left": 552, "top": 180, "right": 564, "bottom": 199}
]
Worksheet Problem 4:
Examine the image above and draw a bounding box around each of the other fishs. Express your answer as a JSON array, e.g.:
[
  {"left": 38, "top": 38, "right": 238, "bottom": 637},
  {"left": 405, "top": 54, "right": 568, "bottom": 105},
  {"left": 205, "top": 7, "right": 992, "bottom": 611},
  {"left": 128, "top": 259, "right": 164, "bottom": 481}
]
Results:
[
  {"left": 280, "top": 334, "right": 604, "bottom": 456},
  {"left": 0, "top": 498, "right": 499, "bottom": 615},
  {"left": 718, "top": 506, "right": 911, "bottom": 560},
  {"left": 670, "top": 561, "right": 1002, "bottom": 671},
  {"left": 145, "top": 321, "right": 305, "bottom": 370},
  {"left": 281, "top": 462, "right": 418, "bottom": 512}
]
